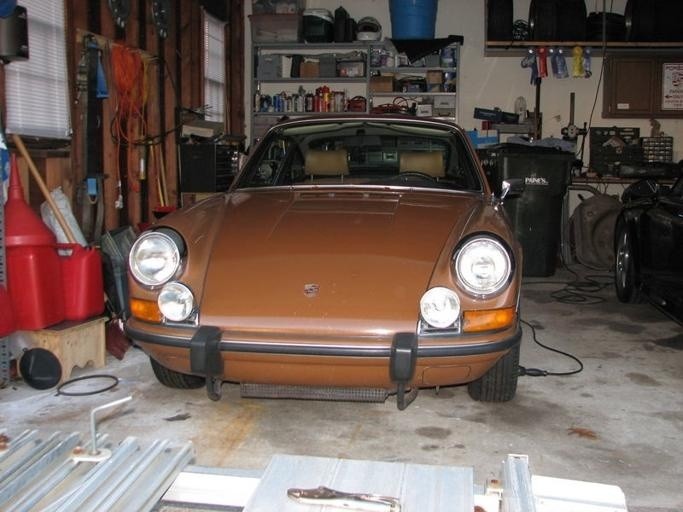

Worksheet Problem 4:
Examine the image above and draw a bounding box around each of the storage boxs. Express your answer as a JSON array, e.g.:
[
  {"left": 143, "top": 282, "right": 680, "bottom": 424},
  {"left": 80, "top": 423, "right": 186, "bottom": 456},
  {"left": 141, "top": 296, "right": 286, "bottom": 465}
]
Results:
[{"left": 247, "top": 14, "right": 303, "bottom": 43}]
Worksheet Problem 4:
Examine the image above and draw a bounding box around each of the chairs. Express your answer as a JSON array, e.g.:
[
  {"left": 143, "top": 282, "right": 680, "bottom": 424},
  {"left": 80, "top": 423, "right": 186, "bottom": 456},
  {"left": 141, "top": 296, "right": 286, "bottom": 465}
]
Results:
[{"left": 299, "top": 150, "right": 447, "bottom": 186}]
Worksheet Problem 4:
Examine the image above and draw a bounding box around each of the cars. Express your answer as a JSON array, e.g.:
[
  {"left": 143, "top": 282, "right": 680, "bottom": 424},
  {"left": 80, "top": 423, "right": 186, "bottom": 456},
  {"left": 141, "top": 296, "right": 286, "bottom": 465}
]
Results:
[
  {"left": 613, "top": 160, "right": 683, "bottom": 329},
  {"left": 122, "top": 115, "right": 526, "bottom": 409}
]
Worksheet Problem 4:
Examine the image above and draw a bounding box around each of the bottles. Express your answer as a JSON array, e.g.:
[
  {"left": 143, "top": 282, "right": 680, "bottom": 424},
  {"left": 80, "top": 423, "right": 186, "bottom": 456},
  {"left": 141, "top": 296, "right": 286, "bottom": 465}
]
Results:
[
  {"left": 255, "top": 136, "right": 262, "bottom": 145},
  {"left": 254, "top": 82, "right": 345, "bottom": 112},
  {"left": 553, "top": 48, "right": 593, "bottom": 80}
]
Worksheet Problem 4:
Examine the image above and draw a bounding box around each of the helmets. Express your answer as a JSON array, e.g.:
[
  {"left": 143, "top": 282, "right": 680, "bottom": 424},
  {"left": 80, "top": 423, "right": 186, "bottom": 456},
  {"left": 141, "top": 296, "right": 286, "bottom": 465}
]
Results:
[{"left": 356, "top": 16, "right": 381, "bottom": 40}]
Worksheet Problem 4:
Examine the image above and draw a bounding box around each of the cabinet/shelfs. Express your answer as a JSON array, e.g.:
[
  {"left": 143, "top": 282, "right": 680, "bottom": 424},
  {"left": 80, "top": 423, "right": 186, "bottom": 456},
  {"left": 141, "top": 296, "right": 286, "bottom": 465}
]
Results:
[
  {"left": 601, "top": 54, "right": 683, "bottom": 119},
  {"left": 249, "top": 34, "right": 462, "bottom": 153}
]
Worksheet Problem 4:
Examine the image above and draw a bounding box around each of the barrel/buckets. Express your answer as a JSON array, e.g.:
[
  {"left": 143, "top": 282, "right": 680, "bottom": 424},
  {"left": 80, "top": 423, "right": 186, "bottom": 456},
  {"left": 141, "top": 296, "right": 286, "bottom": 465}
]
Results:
[
  {"left": 388, "top": 0, "right": 438, "bottom": 40},
  {"left": 55, "top": 243, "right": 104, "bottom": 321}
]
